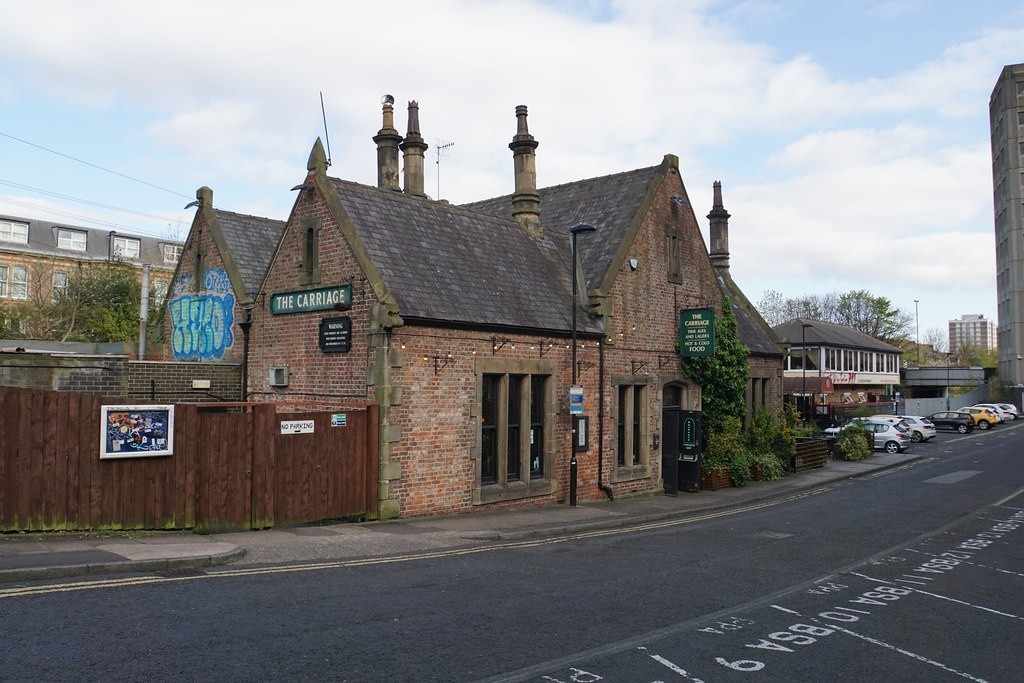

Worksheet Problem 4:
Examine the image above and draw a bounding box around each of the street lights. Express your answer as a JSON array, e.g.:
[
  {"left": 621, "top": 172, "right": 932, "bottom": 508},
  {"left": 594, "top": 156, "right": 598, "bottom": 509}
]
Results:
[
  {"left": 913, "top": 300, "right": 920, "bottom": 367},
  {"left": 947, "top": 353, "right": 954, "bottom": 410},
  {"left": 569, "top": 222, "right": 599, "bottom": 508},
  {"left": 801, "top": 323, "right": 814, "bottom": 428}
]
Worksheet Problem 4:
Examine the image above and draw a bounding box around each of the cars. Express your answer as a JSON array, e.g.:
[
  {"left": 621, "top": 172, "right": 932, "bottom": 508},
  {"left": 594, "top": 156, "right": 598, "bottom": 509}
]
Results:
[
  {"left": 855, "top": 416, "right": 914, "bottom": 443},
  {"left": 993, "top": 403, "right": 1020, "bottom": 421},
  {"left": 870, "top": 415, "right": 937, "bottom": 443},
  {"left": 969, "top": 403, "right": 1010, "bottom": 424},
  {"left": 952, "top": 406, "right": 1001, "bottom": 431},
  {"left": 824, "top": 417, "right": 913, "bottom": 455},
  {"left": 920, "top": 411, "right": 975, "bottom": 434}
]
